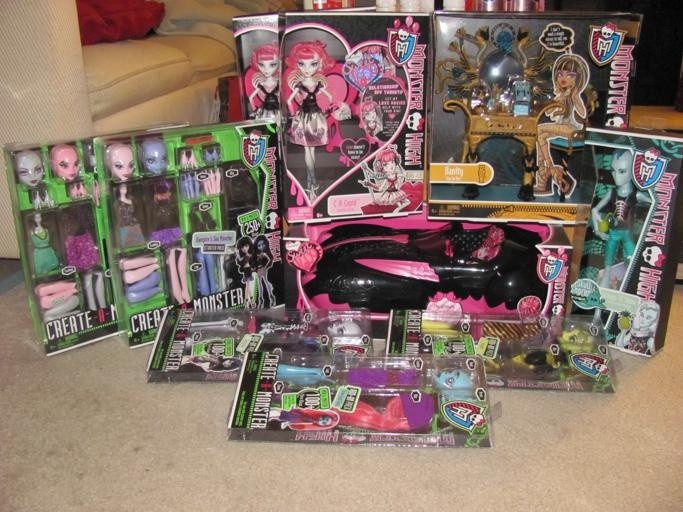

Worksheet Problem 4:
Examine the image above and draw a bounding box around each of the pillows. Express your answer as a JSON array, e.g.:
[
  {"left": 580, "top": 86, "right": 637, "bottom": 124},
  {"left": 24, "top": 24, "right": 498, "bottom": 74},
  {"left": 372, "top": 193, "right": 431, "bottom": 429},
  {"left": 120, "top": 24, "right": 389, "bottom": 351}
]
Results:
[{"left": 77, "top": 0, "right": 164, "bottom": 44}]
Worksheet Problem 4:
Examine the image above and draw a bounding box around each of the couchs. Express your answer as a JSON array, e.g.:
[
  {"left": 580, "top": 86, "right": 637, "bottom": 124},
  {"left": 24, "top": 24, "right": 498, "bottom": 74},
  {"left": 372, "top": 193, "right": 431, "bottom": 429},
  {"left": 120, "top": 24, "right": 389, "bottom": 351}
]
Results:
[{"left": 0, "top": 0, "right": 303, "bottom": 258}]
